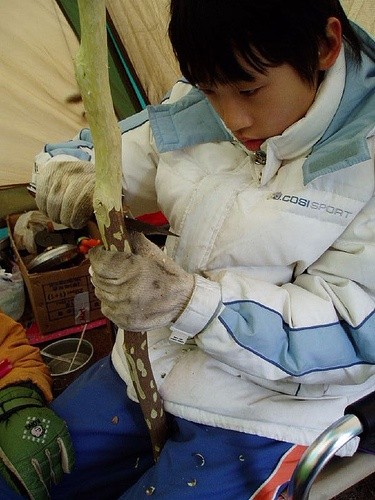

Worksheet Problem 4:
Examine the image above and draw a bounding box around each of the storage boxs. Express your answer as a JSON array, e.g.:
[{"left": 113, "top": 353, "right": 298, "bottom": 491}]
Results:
[{"left": 6, "top": 213, "right": 107, "bottom": 333}]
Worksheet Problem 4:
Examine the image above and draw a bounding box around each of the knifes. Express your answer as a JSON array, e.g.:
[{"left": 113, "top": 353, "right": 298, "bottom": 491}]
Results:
[{"left": 24, "top": 180, "right": 182, "bottom": 239}]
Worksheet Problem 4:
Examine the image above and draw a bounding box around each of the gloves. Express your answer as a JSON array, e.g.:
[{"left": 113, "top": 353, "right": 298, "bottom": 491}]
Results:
[
  {"left": 35, "top": 162, "right": 132, "bottom": 229},
  {"left": 88, "top": 230, "right": 195, "bottom": 332},
  {"left": 0, "top": 385, "right": 75, "bottom": 500}
]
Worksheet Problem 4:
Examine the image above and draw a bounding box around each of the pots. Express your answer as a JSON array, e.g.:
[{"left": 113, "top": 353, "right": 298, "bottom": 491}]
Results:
[{"left": 37, "top": 337, "right": 94, "bottom": 394}]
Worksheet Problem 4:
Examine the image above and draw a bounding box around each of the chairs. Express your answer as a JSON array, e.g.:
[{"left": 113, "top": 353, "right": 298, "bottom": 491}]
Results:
[{"left": 287, "top": 395, "right": 375, "bottom": 500}]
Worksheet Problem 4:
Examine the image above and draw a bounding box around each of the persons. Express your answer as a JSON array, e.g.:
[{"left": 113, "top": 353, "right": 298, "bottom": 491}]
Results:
[
  {"left": 0, "top": 1, "right": 375, "bottom": 500},
  {"left": 0, "top": 313, "right": 76, "bottom": 500}
]
